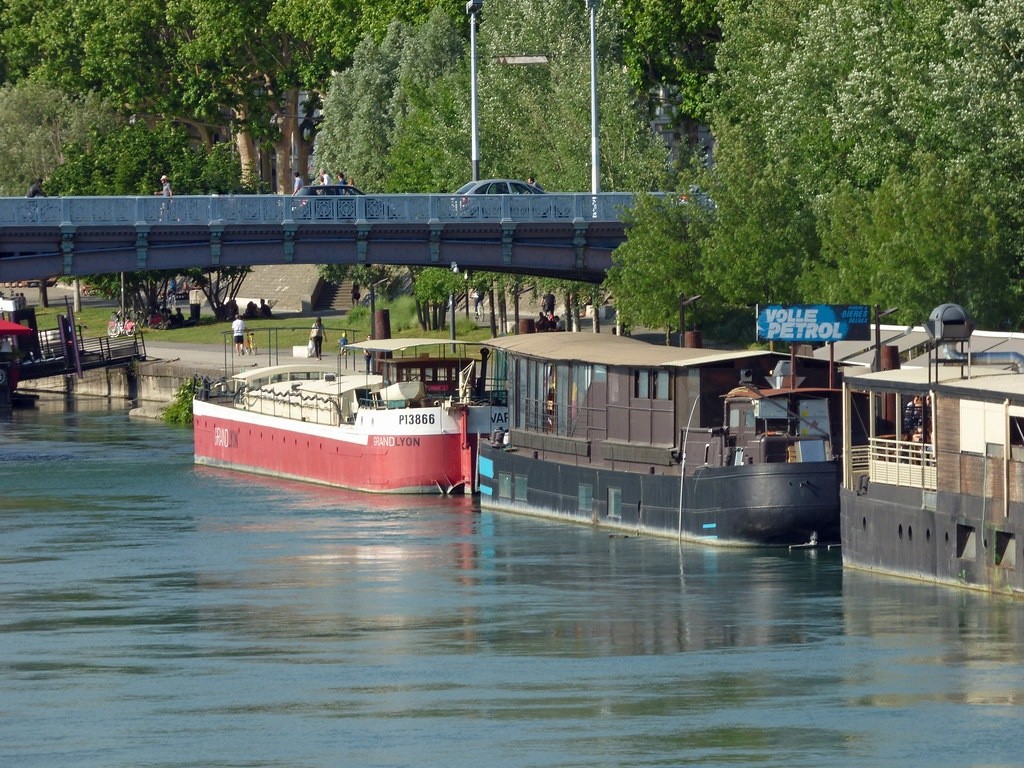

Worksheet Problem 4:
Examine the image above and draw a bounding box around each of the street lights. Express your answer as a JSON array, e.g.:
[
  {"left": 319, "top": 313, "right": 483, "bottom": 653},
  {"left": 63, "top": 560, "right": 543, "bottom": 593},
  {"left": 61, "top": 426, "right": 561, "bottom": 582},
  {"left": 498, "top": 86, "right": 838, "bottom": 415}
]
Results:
[
  {"left": 371, "top": 277, "right": 389, "bottom": 375},
  {"left": 875, "top": 308, "right": 899, "bottom": 436},
  {"left": 680, "top": 295, "right": 702, "bottom": 347},
  {"left": 467, "top": 0, "right": 484, "bottom": 182}
]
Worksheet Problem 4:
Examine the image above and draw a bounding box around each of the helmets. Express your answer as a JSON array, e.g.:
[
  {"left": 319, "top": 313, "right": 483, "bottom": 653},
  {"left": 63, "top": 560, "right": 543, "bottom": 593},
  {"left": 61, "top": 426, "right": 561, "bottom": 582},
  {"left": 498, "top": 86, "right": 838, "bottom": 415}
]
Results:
[{"left": 341, "top": 333, "right": 347, "bottom": 338}]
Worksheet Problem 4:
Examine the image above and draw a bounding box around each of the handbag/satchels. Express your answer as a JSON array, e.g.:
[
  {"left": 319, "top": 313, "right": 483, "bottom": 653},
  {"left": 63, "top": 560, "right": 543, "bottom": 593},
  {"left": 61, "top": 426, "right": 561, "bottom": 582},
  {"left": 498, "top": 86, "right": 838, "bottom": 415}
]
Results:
[
  {"left": 308, "top": 340, "right": 314, "bottom": 352},
  {"left": 310, "top": 329, "right": 320, "bottom": 337}
]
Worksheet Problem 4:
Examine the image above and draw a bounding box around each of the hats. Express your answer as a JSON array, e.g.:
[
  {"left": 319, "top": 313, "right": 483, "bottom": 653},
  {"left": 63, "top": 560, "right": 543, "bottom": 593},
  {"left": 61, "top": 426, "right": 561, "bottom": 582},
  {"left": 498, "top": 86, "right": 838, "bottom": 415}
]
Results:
[{"left": 160, "top": 175, "right": 169, "bottom": 183}]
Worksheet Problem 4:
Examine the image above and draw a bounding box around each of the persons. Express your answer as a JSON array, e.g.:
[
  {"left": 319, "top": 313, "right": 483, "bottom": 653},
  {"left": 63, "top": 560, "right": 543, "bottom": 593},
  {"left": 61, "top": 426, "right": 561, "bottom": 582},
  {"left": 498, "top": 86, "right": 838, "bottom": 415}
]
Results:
[
  {"left": 338, "top": 332, "right": 348, "bottom": 356},
  {"left": 0, "top": 288, "right": 26, "bottom": 310},
  {"left": 534, "top": 289, "right": 592, "bottom": 332},
  {"left": 24, "top": 179, "right": 48, "bottom": 198},
  {"left": 308, "top": 316, "right": 328, "bottom": 360},
  {"left": 147, "top": 275, "right": 197, "bottom": 330},
  {"left": 523, "top": 176, "right": 544, "bottom": 195},
  {"left": 351, "top": 280, "right": 361, "bottom": 309},
  {"left": 231, "top": 314, "right": 248, "bottom": 356},
  {"left": 154, "top": 175, "right": 182, "bottom": 221},
  {"left": 470, "top": 286, "right": 485, "bottom": 319},
  {"left": 290, "top": 168, "right": 354, "bottom": 211},
  {"left": 362, "top": 335, "right": 373, "bottom": 375},
  {"left": 903, "top": 394, "right": 931, "bottom": 467},
  {"left": 225, "top": 299, "right": 272, "bottom": 319}
]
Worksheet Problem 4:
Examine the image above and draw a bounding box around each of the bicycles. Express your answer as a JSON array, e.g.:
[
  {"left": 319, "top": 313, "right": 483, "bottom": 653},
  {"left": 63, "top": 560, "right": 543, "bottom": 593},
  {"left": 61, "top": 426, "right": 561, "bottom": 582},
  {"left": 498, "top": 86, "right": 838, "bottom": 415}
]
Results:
[{"left": 107, "top": 310, "right": 141, "bottom": 337}]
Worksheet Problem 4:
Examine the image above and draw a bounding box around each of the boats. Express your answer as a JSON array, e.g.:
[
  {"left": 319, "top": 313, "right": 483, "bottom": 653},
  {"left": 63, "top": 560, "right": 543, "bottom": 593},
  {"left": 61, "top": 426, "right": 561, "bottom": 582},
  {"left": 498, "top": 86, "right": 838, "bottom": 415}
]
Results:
[
  {"left": 838, "top": 302, "right": 1024, "bottom": 596},
  {"left": 193, "top": 337, "right": 508, "bottom": 494},
  {"left": 475, "top": 330, "right": 871, "bottom": 546}
]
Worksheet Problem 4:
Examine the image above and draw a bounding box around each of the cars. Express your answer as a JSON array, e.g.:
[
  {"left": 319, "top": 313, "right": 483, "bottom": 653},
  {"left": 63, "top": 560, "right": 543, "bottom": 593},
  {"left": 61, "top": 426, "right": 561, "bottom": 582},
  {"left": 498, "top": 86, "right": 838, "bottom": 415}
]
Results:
[
  {"left": 451, "top": 179, "right": 570, "bottom": 218},
  {"left": 291, "top": 185, "right": 400, "bottom": 219}
]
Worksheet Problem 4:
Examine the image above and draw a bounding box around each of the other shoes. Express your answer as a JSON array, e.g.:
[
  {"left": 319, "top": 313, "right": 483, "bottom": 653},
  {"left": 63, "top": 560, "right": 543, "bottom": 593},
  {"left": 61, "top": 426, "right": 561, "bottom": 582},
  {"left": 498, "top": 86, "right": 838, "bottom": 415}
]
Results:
[
  {"left": 241, "top": 349, "right": 244, "bottom": 355},
  {"left": 237, "top": 353, "right": 240, "bottom": 356}
]
